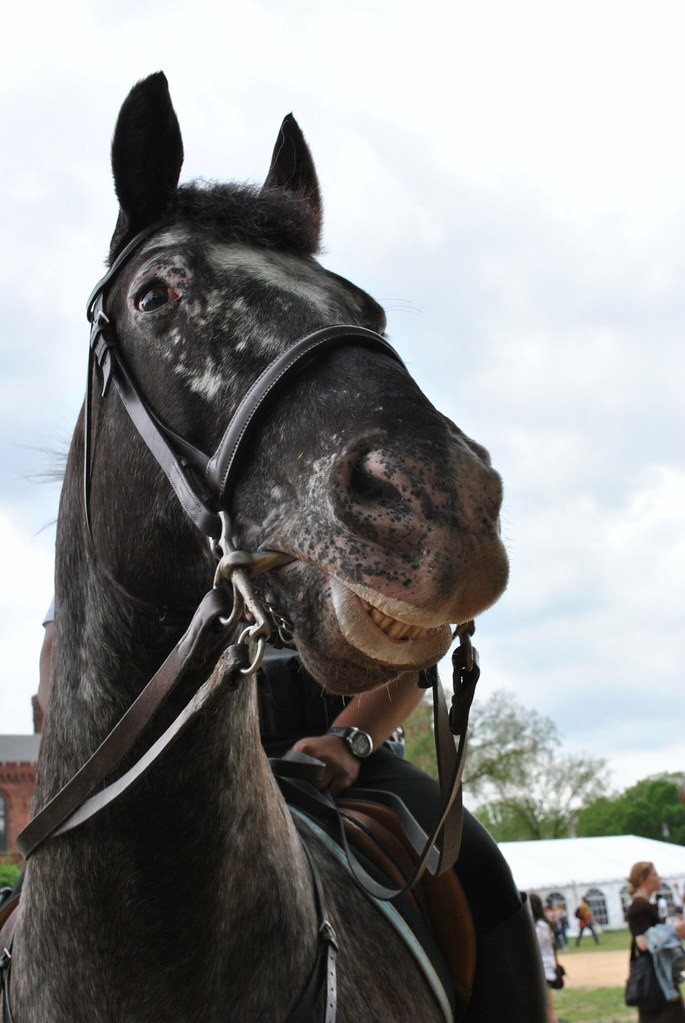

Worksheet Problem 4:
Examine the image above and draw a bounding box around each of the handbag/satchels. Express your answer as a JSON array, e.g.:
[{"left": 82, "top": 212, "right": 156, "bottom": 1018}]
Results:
[
  {"left": 547, "top": 964, "right": 566, "bottom": 990},
  {"left": 622, "top": 954, "right": 663, "bottom": 1007}
]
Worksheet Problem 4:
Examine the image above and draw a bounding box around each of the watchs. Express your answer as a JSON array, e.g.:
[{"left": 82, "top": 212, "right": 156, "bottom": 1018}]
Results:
[{"left": 327, "top": 724, "right": 375, "bottom": 760}]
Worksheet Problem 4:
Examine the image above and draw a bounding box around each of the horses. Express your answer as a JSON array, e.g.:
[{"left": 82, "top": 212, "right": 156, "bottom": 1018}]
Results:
[{"left": 8, "top": 71, "right": 511, "bottom": 1022}]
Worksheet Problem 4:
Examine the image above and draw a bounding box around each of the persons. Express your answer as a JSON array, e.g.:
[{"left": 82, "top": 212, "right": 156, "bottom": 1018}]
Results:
[
  {"left": 625, "top": 862, "right": 685, "bottom": 1023},
  {"left": 574, "top": 897, "right": 602, "bottom": 944},
  {"left": 528, "top": 893, "right": 565, "bottom": 1022},
  {"left": 250, "top": 634, "right": 550, "bottom": 1021}
]
